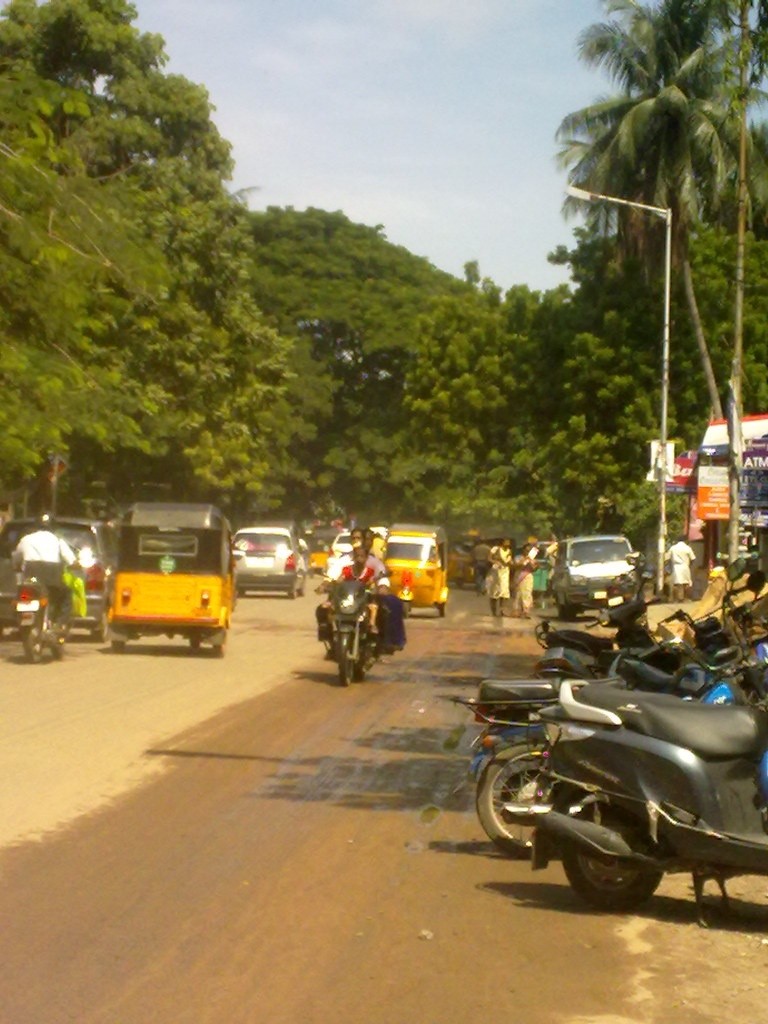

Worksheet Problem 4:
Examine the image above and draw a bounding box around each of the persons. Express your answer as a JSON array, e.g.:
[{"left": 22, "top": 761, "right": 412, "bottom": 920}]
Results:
[
  {"left": 664, "top": 538, "right": 697, "bottom": 601},
  {"left": 11, "top": 512, "right": 79, "bottom": 622},
  {"left": 486, "top": 536, "right": 516, "bottom": 615},
  {"left": 321, "top": 524, "right": 408, "bottom": 652},
  {"left": 512, "top": 543, "right": 536, "bottom": 620},
  {"left": 527, "top": 535, "right": 544, "bottom": 560},
  {"left": 472, "top": 539, "right": 492, "bottom": 596}
]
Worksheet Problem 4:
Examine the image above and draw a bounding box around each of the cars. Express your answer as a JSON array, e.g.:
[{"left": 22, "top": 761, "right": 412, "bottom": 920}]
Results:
[{"left": 0, "top": 514, "right": 116, "bottom": 644}]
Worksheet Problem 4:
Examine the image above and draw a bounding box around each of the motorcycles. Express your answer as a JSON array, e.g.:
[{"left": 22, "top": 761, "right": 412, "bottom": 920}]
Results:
[
  {"left": 524, "top": 543, "right": 553, "bottom": 595},
  {"left": 315, "top": 575, "right": 383, "bottom": 687},
  {"left": 449, "top": 554, "right": 767, "bottom": 923},
  {"left": 12, "top": 572, "right": 78, "bottom": 666},
  {"left": 109, "top": 500, "right": 234, "bottom": 657},
  {"left": 383, "top": 524, "right": 454, "bottom": 617}
]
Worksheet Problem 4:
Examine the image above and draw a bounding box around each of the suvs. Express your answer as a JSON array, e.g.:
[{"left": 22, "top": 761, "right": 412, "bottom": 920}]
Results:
[{"left": 551, "top": 537, "right": 639, "bottom": 618}]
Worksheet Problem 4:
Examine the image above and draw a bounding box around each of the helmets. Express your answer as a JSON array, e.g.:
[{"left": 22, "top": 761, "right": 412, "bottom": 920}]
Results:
[{"left": 35, "top": 511, "right": 56, "bottom": 531}]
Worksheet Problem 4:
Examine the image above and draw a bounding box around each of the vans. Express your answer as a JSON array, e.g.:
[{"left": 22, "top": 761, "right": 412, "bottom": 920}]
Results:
[{"left": 228, "top": 523, "right": 355, "bottom": 597}]
